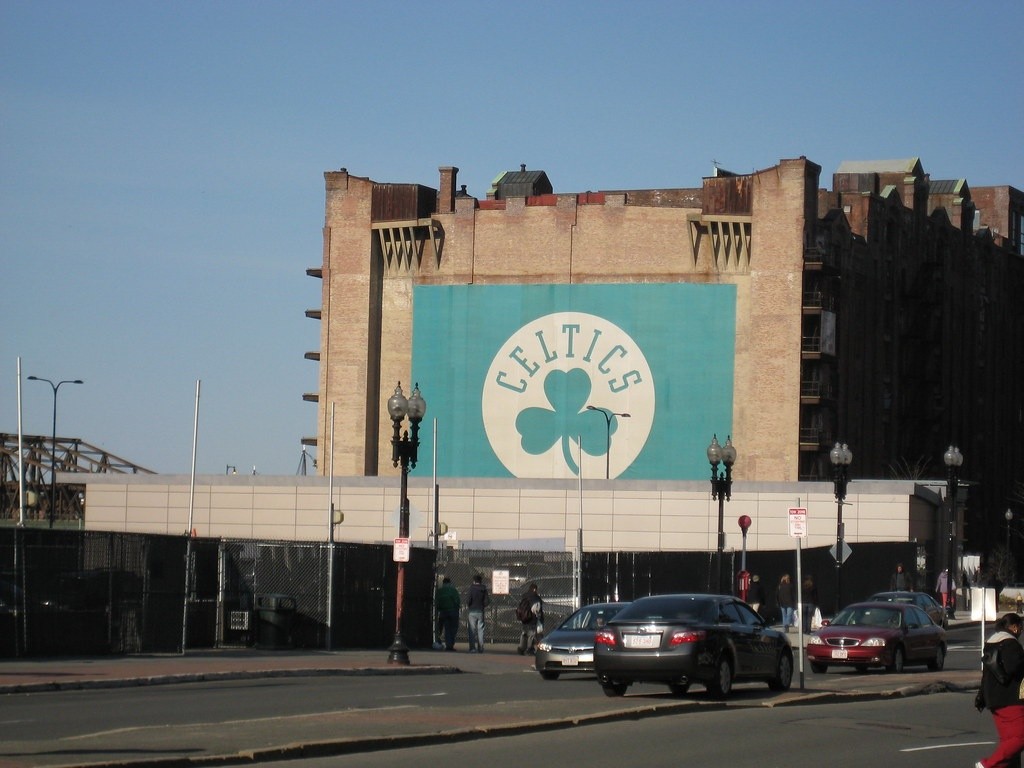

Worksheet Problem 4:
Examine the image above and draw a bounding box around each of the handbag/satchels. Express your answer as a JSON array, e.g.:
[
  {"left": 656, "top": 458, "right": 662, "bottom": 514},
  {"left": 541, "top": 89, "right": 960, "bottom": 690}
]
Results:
[
  {"left": 536, "top": 618, "right": 544, "bottom": 635},
  {"left": 791, "top": 609, "right": 799, "bottom": 627},
  {"left": 975, "top": 687, "right": 987, "bottom": 714},
  {"left": 981, "top": 638, "right": 1024, "bottom": 687},
  {"left": 811, "top": 607, "right": 823, "bottom": 631}
]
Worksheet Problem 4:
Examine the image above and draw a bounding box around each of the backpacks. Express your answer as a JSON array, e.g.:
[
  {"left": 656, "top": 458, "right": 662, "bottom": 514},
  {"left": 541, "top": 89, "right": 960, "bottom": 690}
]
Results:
[{"left": 516, "top": 598, "right": 532, "bottom": 623}]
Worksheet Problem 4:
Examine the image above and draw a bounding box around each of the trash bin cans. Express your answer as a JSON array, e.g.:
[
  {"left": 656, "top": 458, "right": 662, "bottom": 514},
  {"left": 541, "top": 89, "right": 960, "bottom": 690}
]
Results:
[{"left": 255, "top": 595, "right": 297, "bottom": 650}]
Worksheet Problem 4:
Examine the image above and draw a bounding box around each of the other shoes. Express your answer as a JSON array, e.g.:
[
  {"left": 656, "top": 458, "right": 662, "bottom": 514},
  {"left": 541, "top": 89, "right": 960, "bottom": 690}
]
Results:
[
  {"left": 975, "top": 761, "right": 984, "bottom": 768},
  {"left": 784, "top": 624, "right": 789, "bottom": 633},
  {"left": 526, "top": 647, "right": 535, "bottom": 656},
  {"left": 518, "top": 646, "right": 524, "bottom": 656}
]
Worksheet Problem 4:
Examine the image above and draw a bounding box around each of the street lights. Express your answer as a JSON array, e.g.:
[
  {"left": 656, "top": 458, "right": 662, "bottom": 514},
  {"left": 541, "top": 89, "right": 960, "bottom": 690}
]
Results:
[
  {"left": 1005, "top": 507, "right": 1013, "bottom": 585},
  {"left": 829, "top": 441, "right": 853, "bottom": 615},
  {"left": 945, "top": 445, "right": 962, "bottom": 619},
  {"left": 586, "top": 405, "right": 631, "bottom": 479},
  {"left": 27, "top": 376, "right": 83, "bottom": 530},
  {"left": 387, "top": 381, "right": 427, "bottom": 665},
  {"left": 707, "top": 433, "right": 737, "bottom": 591}
]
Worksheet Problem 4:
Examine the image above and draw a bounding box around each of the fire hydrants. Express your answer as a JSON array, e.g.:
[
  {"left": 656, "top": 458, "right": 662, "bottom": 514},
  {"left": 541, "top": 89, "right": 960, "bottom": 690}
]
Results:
[{"left": 1016, "top": 593, "right": 1023, "bottom": 613}]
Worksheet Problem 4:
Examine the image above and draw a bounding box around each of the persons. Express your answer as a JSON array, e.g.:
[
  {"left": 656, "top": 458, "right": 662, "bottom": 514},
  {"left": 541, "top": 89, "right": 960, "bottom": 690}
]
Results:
[
  {"left": 801, "top": 580, "right": 816, "bottom": 635},
  {"left": 467, "top": 575, "right": 490, "bottom": 653},
  {"left": 518, "top": 584, "right": 544, "bottom": 655},
  {"left": 935, "top": 568, "right": 955, "bottom": 607},
  {"left": 975, "top": 613, "right": 1024, "bottom": 768},
  {"left": 436, "top": 578, "right": 460, "bottom": 650},
  {"left": 890, "top": 563, "right": 912, "bottom": 591},
  {"left": 748, "top": 575, "right": 760, "bottom": 613},
  {"left": 780, "top": 574, "right": 792, "bottom": 633}
]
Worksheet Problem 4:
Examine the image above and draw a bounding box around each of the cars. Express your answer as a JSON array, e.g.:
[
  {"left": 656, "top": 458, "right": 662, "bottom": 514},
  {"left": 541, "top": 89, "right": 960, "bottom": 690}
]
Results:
[
  {"left": 535, "top": 602, "right": 632, "bottom": 679},
  {"left": 594, "top": 594, "right": 793, "bottom": 701},
  {"left": 868, "top": 592, "right": 948, "bottom": 630},
  {"left": 805, "top": 601, "right": 948, "bottom": 675}
]
